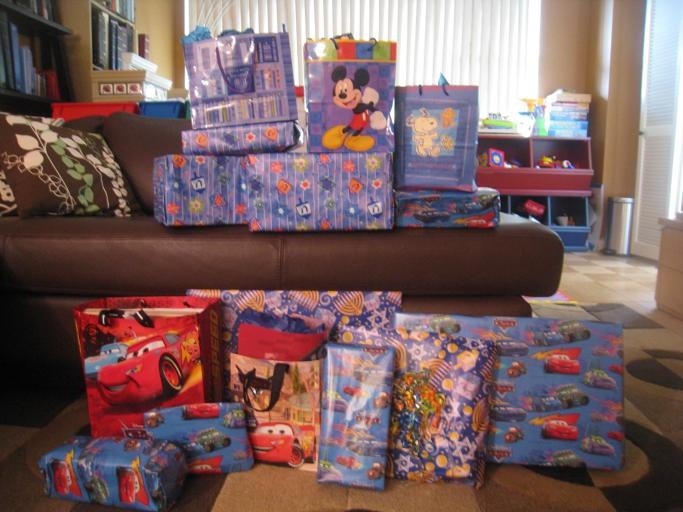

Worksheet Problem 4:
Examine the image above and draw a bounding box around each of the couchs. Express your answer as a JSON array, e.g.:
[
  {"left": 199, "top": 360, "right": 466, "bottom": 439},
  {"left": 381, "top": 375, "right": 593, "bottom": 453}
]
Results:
[{"left": 0, "top": 112, "right": 566, "bottom": 373}]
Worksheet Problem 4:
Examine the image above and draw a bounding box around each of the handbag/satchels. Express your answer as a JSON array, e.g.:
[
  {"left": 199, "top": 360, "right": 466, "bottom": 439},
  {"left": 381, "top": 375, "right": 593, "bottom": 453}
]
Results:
[
  {"left": 72, "top": 296, "right": 221, "bottom": 437},
  {"left": 180, "top": 23, "right": 297, "bottom": 130},
  {"left": 230, "top": 352, "right": 322, "bottom": 473},
  {"left": 396, "top": 84, "right": 479, "bottom": 193},
  {"left": 304, "top": 40, "right": 394, "bottom": 153}
]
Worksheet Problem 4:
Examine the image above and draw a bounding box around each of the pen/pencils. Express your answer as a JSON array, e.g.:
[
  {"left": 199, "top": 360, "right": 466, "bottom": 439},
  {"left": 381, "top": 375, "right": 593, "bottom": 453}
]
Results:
[{"left": 489, "top": 113, "right": 501, "bottom": 120}]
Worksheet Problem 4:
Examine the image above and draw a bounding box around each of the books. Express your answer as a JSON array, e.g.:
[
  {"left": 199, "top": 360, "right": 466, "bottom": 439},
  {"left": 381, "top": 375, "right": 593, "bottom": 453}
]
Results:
[{"left": 0, "top": 0, "right": 150, "bottom": 99}]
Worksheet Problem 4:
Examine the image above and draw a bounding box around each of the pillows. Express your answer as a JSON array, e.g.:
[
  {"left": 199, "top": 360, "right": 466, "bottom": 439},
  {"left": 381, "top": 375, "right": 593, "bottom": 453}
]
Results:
[{"left": 0, "top": 112, "right": 147, "bottom": 223}]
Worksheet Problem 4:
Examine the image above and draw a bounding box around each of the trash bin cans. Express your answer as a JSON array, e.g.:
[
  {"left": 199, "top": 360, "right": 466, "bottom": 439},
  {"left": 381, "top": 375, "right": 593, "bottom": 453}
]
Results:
[{"left": 608, "top": 196, "right": 634, "bottom": 255}]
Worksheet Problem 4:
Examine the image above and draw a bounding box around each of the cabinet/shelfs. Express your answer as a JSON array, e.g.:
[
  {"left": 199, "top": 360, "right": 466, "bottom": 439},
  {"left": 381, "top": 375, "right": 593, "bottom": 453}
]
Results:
[
  {"left": 0, "top": 0, "right": 139, "bottom": 115},
  {"left": 476, "top": 131, "right": 596, "bottom": 250}
]
